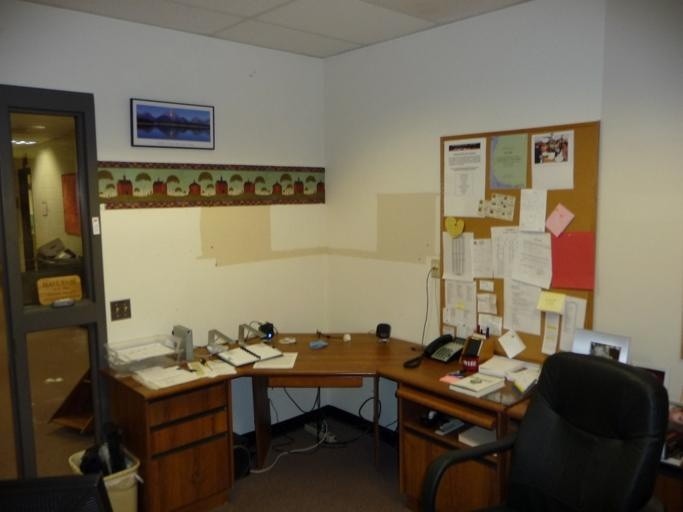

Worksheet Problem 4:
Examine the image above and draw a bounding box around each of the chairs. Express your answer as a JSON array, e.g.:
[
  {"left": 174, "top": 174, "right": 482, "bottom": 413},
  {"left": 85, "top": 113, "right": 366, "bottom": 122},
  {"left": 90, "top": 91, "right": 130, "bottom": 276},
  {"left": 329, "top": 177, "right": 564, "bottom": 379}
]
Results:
[
  {"left": 0, "top": 473, "right": 112, "bottom": 512},
  {"left": 421, "top": 351, "right": 669, "bottom": 511}
]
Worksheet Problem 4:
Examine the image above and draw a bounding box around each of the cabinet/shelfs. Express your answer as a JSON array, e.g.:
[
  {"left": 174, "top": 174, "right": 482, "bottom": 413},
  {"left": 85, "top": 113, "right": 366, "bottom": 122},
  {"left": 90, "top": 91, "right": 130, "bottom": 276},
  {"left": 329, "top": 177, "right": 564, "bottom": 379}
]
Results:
[{"left": 98, "top": 332, "right": 551, "bottom": 512}]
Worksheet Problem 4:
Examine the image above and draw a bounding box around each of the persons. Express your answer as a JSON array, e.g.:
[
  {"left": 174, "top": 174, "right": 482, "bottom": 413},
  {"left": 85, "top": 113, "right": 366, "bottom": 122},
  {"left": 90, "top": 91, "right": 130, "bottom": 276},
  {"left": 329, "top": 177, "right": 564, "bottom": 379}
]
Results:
[
  {"left": 533, "top": 136, "right": 568, "bottom": 163},
  {"left": 590, "top": 343, "right": 616, "bottom": 361}
]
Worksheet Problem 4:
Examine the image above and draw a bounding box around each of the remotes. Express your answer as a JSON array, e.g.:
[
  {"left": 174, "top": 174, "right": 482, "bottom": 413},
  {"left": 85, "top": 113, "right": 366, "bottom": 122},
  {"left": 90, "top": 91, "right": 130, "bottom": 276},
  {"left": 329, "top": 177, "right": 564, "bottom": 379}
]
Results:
[{"left": 435, "top": 419, "right": 466, "bottom": 437}]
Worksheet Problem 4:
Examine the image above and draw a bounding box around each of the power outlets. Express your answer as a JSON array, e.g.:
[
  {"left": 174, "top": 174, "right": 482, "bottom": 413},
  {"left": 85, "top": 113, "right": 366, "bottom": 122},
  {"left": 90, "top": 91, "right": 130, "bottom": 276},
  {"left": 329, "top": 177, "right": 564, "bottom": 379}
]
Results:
[{"left": 430, "top": 257, "right": 442, "bottom": 278}]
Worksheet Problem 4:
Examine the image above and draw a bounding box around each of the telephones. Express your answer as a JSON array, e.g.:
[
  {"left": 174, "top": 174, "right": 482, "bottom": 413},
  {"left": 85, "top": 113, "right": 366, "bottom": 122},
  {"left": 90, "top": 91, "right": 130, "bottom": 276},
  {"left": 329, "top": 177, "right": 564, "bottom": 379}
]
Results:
[{"left": 425, "top": 334, "right": 466, "bottom": 364}]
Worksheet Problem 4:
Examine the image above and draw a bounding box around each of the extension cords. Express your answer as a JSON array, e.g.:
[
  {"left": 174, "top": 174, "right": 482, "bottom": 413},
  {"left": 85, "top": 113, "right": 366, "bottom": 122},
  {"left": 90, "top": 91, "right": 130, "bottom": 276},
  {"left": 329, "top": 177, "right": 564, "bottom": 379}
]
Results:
[{"left": 304, "top": 422, "right": 336, "bottom": 444}]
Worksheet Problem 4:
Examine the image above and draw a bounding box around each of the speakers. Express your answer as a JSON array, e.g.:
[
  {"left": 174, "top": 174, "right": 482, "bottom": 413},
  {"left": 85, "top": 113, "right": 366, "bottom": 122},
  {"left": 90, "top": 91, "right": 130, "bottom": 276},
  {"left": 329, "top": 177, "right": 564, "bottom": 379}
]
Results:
[
  {"left": 259, "top": 323, "right": 274, "bottom": 340},
  {"left": 376, "top": 324, "right": 392, "bottom": 339}
]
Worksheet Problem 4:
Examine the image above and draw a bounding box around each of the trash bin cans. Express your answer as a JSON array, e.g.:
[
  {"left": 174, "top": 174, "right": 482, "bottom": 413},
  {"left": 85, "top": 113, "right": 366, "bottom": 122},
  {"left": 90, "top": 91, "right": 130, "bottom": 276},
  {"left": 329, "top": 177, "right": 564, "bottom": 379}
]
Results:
[{"left": 68, "top": 447, "right": 140, "bottom": 512}]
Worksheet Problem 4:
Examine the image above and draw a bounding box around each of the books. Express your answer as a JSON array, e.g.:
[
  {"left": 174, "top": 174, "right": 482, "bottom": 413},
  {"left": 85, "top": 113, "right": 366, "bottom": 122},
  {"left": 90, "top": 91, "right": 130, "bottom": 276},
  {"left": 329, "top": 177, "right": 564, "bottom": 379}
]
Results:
[
  {"left": 478, "top": 354, "right": 526, "bottom": 378},
  {"left": 215, "top": 340, "right": 283, "bottom": 368},
  {"left": 449, "top": 372, "right": 505, "bottom": 401},
  {"left": 188, "top": 357, "right": 238, "bottom": 382},
  {"left": 459, "top": 423, "right": 499, "bottom": 458}
]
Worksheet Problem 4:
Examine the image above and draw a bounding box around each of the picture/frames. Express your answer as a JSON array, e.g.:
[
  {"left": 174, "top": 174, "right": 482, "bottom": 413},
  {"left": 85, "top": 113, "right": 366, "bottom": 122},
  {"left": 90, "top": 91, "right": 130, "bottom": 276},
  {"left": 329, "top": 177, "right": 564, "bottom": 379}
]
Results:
[{"left": 130, "top": 98, "right": 215, "bottom": 150}]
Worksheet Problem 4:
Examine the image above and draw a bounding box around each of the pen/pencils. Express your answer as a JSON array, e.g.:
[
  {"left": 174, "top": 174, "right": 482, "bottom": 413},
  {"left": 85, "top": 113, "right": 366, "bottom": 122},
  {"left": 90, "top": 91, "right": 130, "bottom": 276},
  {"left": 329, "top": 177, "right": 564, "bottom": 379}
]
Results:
[{"left": 477, "top": 325, "right": 489, "bottom": 338}]
